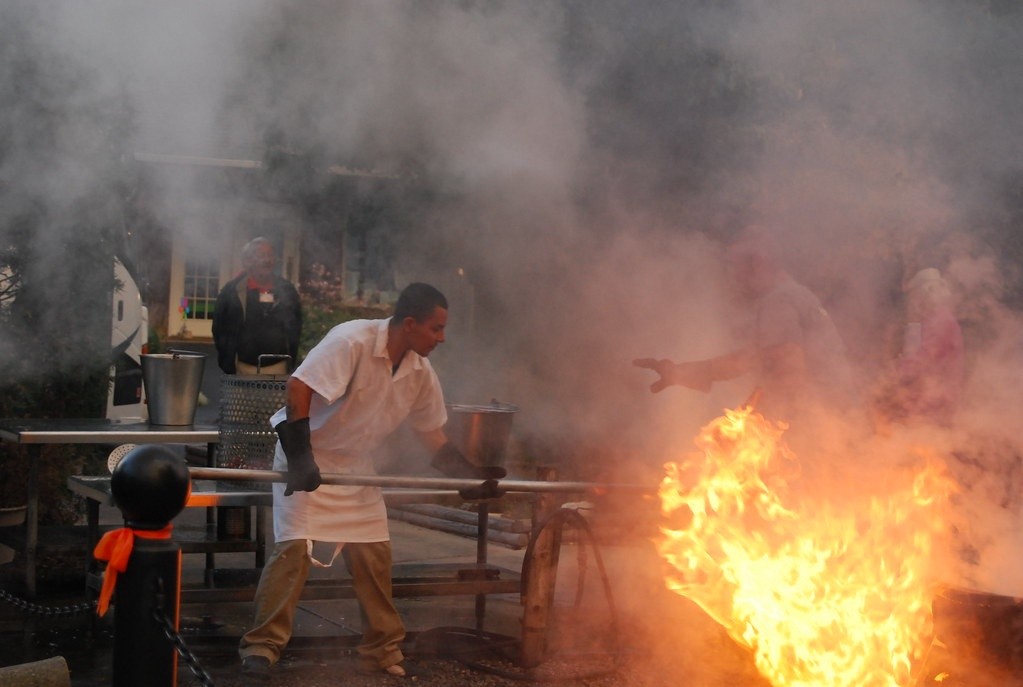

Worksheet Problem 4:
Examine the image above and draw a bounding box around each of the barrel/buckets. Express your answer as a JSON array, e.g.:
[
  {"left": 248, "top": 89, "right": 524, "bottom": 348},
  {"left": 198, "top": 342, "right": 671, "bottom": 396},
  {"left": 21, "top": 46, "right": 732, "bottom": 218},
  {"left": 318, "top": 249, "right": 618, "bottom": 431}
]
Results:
[
  {"left": 139, "top": 347, "right": 208, "bottom": 425},
  {"left": 452, "top": 398, "right": 521, "bottom": 467}
]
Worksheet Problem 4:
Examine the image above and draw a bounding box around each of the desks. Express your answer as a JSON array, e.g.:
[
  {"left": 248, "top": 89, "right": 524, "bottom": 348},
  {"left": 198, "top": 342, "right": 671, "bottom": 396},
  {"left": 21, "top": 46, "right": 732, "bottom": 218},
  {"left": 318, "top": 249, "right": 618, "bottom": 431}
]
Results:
[
  {"left": 0, "top": 419, "right": 279, "bottom": 570},
  {"left": 65, "top": 468, "right": 568, "bottom": 670}
]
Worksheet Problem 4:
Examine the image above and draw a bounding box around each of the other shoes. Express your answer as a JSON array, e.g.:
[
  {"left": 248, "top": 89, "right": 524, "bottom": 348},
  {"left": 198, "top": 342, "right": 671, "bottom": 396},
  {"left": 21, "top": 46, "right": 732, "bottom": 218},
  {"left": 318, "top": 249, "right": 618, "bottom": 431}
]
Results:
[
  {"left": 385, "top": 659, "right": 435, "bottom": 682},
  {"left": 242, "top": 656, "right": 273, "bottom": 680}
]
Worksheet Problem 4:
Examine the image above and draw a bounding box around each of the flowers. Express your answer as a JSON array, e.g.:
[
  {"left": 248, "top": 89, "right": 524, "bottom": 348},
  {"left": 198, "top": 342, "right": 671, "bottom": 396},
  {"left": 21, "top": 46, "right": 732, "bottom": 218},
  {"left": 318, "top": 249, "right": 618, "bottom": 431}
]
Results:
[{"left": 296, "top": 263, "right": 359, "bottom": 364}]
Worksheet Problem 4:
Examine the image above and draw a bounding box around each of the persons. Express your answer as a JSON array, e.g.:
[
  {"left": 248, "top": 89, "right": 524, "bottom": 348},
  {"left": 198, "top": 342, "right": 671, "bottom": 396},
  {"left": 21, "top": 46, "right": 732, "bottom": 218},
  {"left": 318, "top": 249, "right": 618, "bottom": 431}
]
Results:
[
  {"left": 239, "top": 282, "right": 509, "bottom": 680},
  {"left": 212, "top": 237, "right": 302, "bottom": 460},
  {"left": 632, "top": 224, "right": 858, "bottom": 454},
  {"left": 878, "top": 268, "right": 1012, "bottom": 427}
]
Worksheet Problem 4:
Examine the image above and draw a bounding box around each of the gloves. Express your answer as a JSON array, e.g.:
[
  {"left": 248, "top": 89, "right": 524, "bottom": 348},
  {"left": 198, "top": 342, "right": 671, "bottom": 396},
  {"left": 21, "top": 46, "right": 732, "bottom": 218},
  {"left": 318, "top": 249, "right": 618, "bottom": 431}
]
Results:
[
  {"left": 430, "top": 439, "right": 507, "bottom": 500},
  {"left": 276, "top": 418, "right": 321, "bottom": 497}
]
